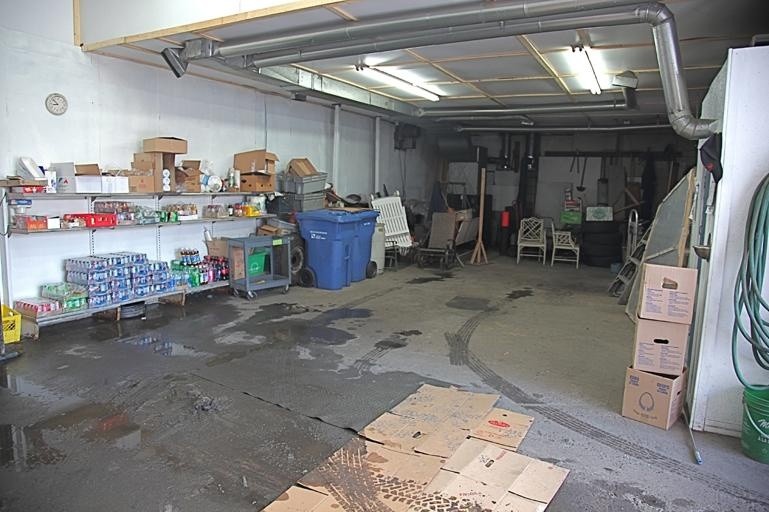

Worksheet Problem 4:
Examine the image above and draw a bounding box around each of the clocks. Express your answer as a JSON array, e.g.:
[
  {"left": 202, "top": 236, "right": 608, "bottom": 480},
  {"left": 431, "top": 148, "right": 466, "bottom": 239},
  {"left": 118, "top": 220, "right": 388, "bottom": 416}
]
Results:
[{"left": 45, "top": 93, "right": 68, "bottom": 116}]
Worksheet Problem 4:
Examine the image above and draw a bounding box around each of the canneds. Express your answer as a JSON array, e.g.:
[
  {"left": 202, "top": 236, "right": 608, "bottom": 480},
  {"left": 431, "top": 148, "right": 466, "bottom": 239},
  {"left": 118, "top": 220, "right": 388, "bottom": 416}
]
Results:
[{"left": 15, "top": 252, "right": 175, "bottom": 316}]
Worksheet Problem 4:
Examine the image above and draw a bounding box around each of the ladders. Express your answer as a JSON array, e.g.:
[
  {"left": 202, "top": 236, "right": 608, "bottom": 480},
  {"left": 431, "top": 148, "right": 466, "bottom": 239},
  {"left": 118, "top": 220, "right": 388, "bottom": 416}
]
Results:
[{"left": 607, "top": 220, "right": 653, "bottom": 305}]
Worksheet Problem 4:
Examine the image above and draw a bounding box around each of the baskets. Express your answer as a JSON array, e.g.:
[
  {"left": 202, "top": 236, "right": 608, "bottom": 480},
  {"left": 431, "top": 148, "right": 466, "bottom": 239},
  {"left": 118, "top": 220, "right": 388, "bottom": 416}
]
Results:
[
  {"left": 64, "top": 213, "right": 116, "bottom": 227},
  {"left": 1, "top": 304, "right": 22, "bottom": 344}
]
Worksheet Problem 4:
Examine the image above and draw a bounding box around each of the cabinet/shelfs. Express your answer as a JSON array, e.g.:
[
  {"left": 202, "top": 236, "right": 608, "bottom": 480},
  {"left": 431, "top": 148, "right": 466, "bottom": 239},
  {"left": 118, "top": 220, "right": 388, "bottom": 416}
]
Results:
[{"left": 0, "top": 187, "right": 277, "bottom": 327}]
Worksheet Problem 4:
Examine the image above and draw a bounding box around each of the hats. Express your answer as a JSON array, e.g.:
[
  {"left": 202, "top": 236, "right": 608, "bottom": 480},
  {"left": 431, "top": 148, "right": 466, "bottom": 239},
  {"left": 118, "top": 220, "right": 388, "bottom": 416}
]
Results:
[{"left": 700, "top": 132, "right": 723, "bottom": 184}]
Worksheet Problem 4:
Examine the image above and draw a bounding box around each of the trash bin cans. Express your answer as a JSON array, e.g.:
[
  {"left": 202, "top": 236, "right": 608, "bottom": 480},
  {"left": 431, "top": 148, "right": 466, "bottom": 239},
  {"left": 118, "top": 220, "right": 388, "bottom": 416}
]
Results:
[{"left": 294, "top": 206, "right": 380, "bottom": 290}]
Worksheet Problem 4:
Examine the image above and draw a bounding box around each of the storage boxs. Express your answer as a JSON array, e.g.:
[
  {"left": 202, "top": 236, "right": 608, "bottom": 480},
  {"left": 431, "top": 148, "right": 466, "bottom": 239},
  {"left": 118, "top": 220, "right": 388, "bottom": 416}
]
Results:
[
  {"left": 233, "top": 149, "right": 328, "bottom": 212},
  {"left": 50, "top": 136, "right": 201, "bottom": 193},
  {"left": 621, "top": 262, "right": 698, "bottom": 431}
]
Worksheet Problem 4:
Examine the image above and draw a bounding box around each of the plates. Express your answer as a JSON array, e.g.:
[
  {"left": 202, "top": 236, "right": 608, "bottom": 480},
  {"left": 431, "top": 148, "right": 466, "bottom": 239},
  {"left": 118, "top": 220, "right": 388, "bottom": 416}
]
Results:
[{"left": 208, "top": 176, "right": 222, "bottom": 192}]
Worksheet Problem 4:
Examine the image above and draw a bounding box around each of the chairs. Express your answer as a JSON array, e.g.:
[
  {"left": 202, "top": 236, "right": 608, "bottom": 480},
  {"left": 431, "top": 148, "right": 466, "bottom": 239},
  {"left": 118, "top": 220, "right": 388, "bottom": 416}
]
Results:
[
  {"left": 516, "top": 216, "right": 579, "bottom": 268},
  {"left": 368, "top": 189, "right": 419, "bottom": 271},
  {"left": 412, "top": 213, "right": 458, "bottom": 269}
]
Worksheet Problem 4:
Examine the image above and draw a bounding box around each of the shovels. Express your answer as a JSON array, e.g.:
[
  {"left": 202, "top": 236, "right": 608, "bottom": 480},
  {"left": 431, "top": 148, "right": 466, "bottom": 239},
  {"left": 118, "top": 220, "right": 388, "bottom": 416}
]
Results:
[{"left": 576, "top": 158, "right": 588, "bottom": 192}]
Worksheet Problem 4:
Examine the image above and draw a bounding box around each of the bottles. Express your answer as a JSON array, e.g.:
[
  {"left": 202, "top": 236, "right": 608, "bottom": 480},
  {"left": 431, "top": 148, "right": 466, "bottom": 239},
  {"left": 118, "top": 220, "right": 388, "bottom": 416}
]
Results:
[
  {"left": 171, "top": 246, "right": 230, "bottom": 288},
  {"left": 206, "top": 204, "right": 242, "bottom": 219},
  {"left": 227, "top": 167, "right": 241, "bottom": 191}
]
